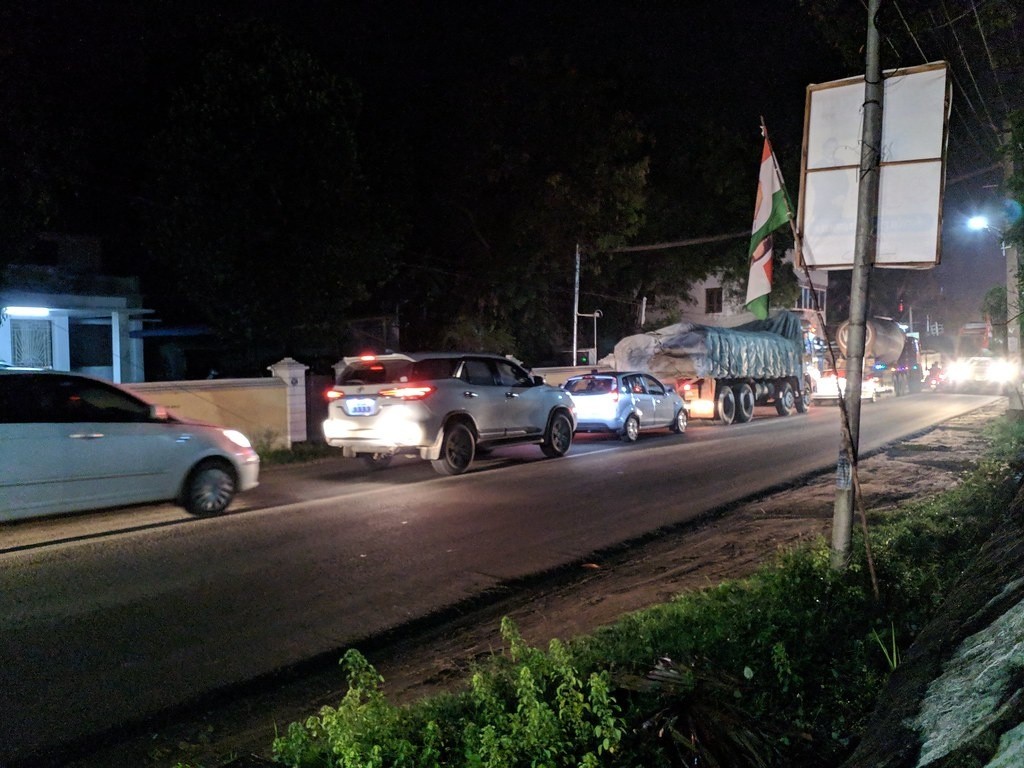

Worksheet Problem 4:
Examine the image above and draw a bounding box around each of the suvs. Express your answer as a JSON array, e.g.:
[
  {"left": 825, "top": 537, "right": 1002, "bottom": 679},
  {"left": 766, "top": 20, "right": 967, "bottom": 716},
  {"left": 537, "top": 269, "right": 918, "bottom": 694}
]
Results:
[{"left": 324, "top": 348, "right": 578, "bottom": 477}]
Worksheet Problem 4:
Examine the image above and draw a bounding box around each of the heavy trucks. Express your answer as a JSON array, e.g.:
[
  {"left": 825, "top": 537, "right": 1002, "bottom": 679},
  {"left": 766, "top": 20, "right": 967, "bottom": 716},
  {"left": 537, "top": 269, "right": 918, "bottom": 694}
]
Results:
[{"left": 615, "top": 310, "right": 827, "bottom": 424}]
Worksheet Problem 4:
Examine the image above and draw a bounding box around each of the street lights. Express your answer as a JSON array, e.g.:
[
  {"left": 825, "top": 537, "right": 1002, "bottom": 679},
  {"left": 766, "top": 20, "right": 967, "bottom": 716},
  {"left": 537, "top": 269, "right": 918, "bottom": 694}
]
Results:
[{"left": 899, "top": 300, "right": 913, "bottom": 335}]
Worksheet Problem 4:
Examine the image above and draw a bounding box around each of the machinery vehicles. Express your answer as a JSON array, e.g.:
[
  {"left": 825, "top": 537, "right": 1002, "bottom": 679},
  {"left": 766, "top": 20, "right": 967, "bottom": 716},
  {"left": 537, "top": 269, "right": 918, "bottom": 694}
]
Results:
[{"left": 811, "top": 313, "right": 923, "bottom": 406}]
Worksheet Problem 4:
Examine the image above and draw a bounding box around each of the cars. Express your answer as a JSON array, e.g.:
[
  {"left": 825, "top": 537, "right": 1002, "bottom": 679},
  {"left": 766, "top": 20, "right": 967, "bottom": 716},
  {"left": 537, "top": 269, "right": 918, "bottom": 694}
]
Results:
[
  {"left": 563, "top": 372, "right": 690, "bottom": 442},
  {"left": 0, "top": 363, "right": 261, "bottom": 517}
]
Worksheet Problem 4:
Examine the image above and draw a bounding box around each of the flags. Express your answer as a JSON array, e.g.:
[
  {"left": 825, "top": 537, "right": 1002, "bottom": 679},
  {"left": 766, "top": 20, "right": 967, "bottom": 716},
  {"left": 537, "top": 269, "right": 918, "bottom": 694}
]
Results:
[
  {"left": 979, "top": 317, "right": 994, "bottom": 356},
  {"left": 743, "top": 129, "right": 795, "bottom": 322}
]
